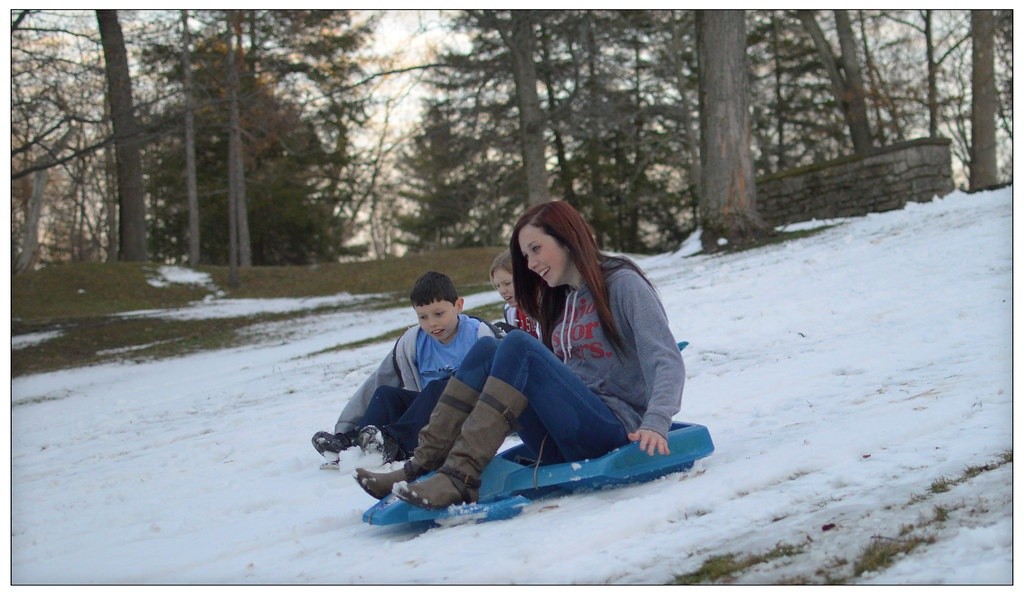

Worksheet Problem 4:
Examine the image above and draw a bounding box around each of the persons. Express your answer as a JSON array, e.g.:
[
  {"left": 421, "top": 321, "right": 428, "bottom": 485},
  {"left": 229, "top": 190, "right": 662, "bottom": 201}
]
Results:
[{"left": 312, "top": 201, "right": 685, "bottom": 512}]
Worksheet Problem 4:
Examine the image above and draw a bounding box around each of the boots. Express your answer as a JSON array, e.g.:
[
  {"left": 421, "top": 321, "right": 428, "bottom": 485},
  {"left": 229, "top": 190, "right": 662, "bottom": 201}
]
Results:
[
  {"left": 391, "top": 376, "right": 529, "bottom": 509},
  {"left": 353, "top": 378, "right": 480, "bottom": 501}
]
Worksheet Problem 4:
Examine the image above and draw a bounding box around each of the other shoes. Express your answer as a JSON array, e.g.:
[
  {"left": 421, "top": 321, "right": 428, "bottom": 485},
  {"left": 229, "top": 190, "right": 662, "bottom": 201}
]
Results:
[
  {"left": 312, "top": 429, "right": 355, "bottom": 465},
  {"left": 356, "top": 422, "right": 408, "bottom": 463}
]
